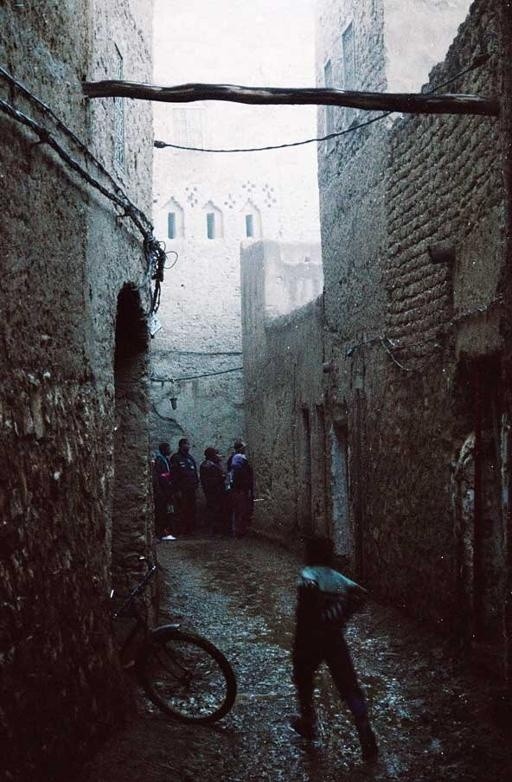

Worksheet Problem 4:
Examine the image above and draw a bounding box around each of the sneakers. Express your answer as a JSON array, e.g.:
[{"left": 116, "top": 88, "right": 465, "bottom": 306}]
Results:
[
  {"left": 161, "top": 534, "right": 177, "bottom": 541},
  {"left": 291, "top": 716, "right": 313, "bottom": 737},
  {"left": 360, "top": 730, "right": 377, "bottom": 762}
]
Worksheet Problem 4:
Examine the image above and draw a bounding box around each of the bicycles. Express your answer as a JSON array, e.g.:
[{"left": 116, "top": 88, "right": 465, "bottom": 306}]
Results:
[{"left": 109, "top": 555, "right": 237, "bottom": 725}]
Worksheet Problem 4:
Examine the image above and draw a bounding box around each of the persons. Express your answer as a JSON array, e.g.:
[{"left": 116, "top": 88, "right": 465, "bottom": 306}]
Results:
[
  {"left": 170, "top": 438, "right": 201, "bottom": 535},
  {"left": 152, "top": 442, "right": 176, "bottom": 541},
  {"left": 227, "top": 439, "right": 250, "bottom": 537},
  {"left": 201, "top": 447, "right": 229, "bottom": 535},
  {"left": 288, "top": 535, "right": 378, "bottom": 765}
]
